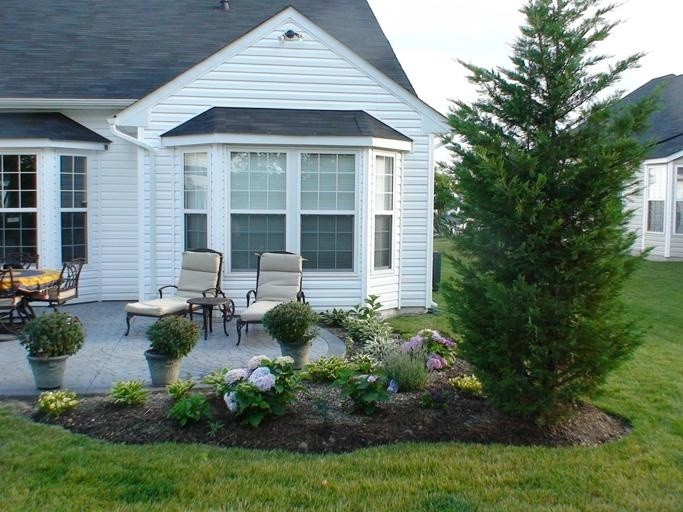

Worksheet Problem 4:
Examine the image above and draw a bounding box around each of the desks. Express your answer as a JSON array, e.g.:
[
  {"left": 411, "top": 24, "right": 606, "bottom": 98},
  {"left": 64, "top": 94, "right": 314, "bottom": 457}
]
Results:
[{"left": 186, "top": 297, "right": 230, "bottom": 341}]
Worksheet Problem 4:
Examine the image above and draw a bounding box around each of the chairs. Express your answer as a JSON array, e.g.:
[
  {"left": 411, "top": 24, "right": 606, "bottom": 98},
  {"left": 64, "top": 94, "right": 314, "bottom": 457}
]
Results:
[
  {"left": 0, "top": 252, "right": 86, "bottom": 336},
  {"left": 234, "top": 249, "right": 306, "bottom": 347},
  {"left": 121, "top": 248, "right": 223, "bottom": 341}
]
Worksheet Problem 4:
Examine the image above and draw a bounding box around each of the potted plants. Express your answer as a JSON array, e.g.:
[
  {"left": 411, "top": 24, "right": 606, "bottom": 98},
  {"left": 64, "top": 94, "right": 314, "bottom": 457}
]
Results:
[
  {"left": 142, "top": 315, "right": 199, "bottom": 386},
  {"left": 260, "top": 299, "right": 320, "bottom": 370},
  {"left": 16, "top": 311, "right": 86, "bottom": 389}
]
